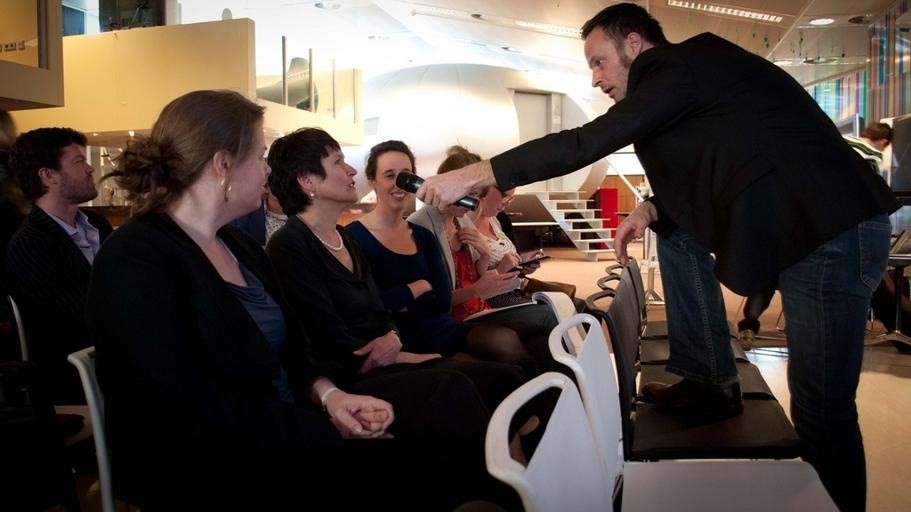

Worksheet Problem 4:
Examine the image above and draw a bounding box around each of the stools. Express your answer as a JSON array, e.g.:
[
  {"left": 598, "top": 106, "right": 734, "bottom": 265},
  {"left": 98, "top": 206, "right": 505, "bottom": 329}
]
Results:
[{"left": 863, "top": 254, "right": 911, "bottom": 347}]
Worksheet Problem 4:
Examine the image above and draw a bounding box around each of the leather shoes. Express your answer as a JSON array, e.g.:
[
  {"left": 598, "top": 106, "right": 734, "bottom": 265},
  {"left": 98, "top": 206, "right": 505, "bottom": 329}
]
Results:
[{"left": 641, "top": 374, "right": 748, "bottom": 416}]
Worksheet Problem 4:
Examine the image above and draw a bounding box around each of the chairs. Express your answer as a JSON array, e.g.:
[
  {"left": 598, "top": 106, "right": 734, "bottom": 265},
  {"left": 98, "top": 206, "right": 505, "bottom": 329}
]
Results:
[
  {"left": 485, "top": 256, "right": 840, "bottom": 511},
  {"left": 65, "top": 346, "right": 124, "bottom": 510}
]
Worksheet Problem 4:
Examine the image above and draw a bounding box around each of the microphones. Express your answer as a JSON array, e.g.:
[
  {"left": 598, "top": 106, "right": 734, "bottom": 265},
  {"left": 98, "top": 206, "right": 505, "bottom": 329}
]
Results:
[{"left": 396, "top": 170, "right": 480, "bottom": 212}]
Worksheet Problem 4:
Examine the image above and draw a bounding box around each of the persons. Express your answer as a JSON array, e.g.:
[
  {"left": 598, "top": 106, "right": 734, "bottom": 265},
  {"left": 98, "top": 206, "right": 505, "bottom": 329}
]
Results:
[
  {"left": 416, "top": 2, "right": 906, "bottom": 512},
  {"left": 736, "top": 120, "right": 911, "bottom": 354},
  {"left": 1, "top": 90, "right": 604, "bottom": 512}
]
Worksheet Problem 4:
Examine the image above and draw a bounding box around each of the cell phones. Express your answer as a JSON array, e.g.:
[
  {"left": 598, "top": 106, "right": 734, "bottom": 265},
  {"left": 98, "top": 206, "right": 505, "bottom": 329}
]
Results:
[
  {"left": 521, "top": 254, "right": 553, "bottom": 268},
  {"left": 506, "top": 264, "right": 523, "bottom": 279}
]
